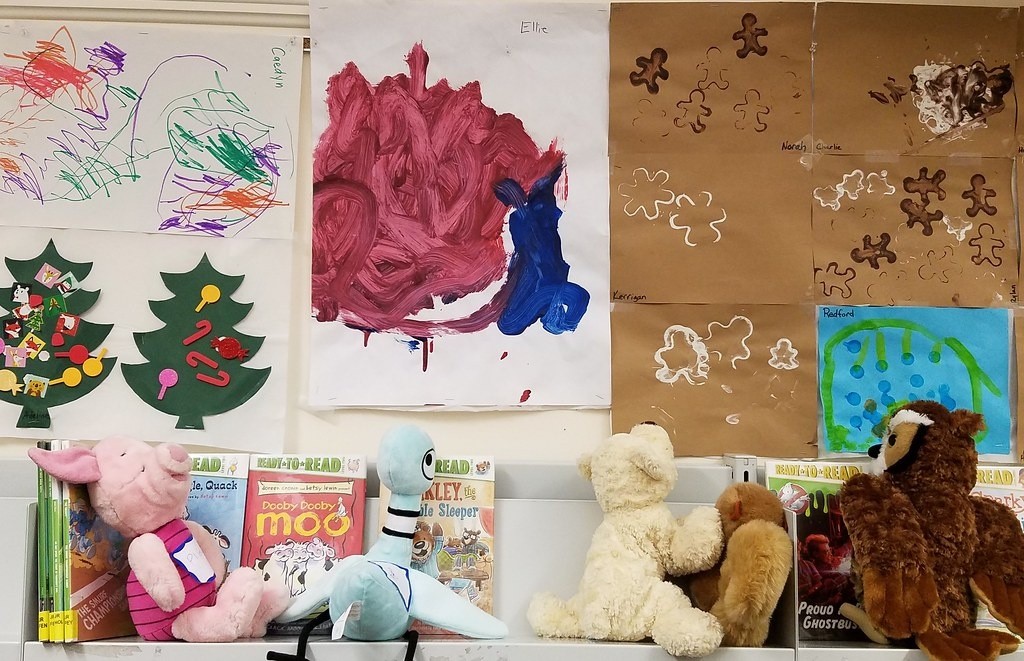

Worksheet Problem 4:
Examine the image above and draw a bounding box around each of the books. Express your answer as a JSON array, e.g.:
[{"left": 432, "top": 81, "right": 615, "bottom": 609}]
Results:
[
  {"left": 964, "top": 466, "right": 1024, "bottom": 643},
  {"left": 378, "top": 456, "right": 496, "bottom": 639},
  {"left": 179, "top": 452, "right": 250, "bottom": 575},
  {"left": 241, "top": 453, "right": 368, "bottom": 636},
  {"left": 34, "top": 440, "right": 137, "bottom": 644},
  {"left": 764, "top": 458, "right": 872, "bottom": 643}
]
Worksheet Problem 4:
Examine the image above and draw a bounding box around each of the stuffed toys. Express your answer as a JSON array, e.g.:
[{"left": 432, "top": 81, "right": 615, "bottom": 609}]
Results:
[
  {"left": 690, "top": 481, "right": 793, "bottom": 648},
  {"left": 273, "top": 422, "right": 508, "bottom": 641},
  {"left": 838, "top": 400, "right": 1024, "bottom": 661},
  {"left": 528, "top": 421, "right": 723, "bottom": 658},
  {"left": 27, "top": 439, "right": 289, "bottom": 643}
]
